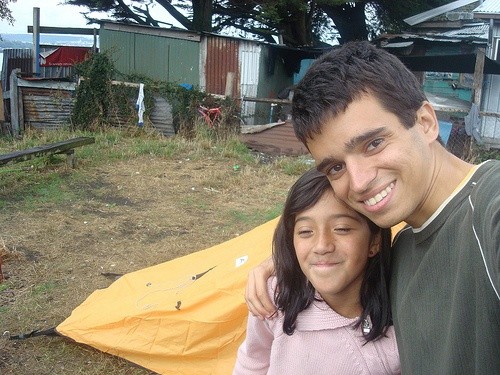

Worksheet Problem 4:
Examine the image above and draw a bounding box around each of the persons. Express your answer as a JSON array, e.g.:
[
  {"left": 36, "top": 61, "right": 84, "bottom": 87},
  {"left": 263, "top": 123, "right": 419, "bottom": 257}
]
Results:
[
  {"left": 245, "top": 40, "right": 500, "bottom": 375},
  {"left": 233, "top": 166, "right": 401, "bottom": 375}
]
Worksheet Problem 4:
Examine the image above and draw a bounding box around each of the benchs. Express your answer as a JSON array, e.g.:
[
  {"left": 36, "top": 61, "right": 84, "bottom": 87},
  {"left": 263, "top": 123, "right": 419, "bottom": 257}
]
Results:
[{"left": 0, "top": 136, "right": 95, "bottom": 170}]
